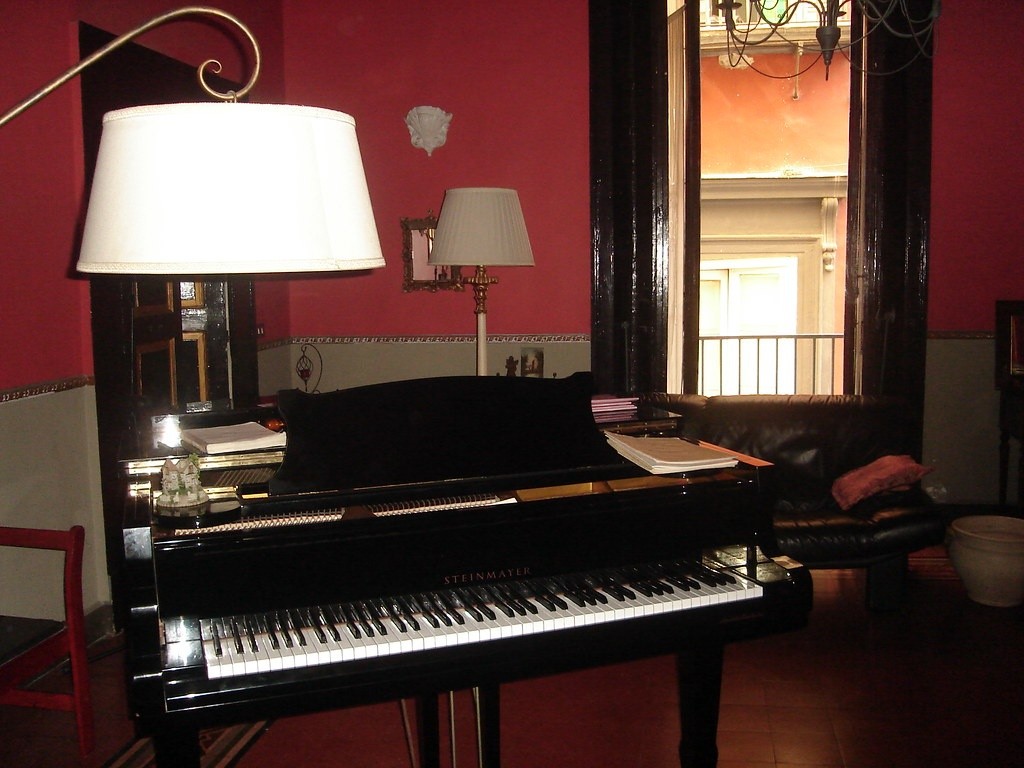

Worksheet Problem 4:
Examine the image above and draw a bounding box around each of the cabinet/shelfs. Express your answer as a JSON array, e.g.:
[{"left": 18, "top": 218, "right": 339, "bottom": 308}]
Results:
[{"left": 993, "top": 299, "right": 1024, "bottom": 519}]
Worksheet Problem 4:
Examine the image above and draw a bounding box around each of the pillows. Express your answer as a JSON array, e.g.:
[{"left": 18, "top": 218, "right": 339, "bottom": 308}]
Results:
[{"left": 830, "top": 454, "right": 933, "bottom": 510}]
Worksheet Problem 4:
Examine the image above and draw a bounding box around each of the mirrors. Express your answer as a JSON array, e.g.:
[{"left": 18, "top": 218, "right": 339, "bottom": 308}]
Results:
[{"left": 401, "top": 207, "right": 465, "bottom": 291}]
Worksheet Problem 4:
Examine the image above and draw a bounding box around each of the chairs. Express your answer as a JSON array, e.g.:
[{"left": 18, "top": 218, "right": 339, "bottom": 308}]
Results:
[{"left": 0, "top": 526, "right": 97, "bottom": 761}]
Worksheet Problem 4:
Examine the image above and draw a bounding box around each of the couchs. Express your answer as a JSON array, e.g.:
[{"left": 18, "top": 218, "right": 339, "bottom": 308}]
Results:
[{"left": 633, "top": 392, "right": 945, "bottom": 612}]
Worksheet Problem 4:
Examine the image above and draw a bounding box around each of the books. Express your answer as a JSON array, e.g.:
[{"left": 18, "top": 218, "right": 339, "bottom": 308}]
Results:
[
  {"left": 592, "top": 392, "right": 739, "bottom": 475},
  {"left": 181, "top": 422, "right": 287, "bottom": 455}
]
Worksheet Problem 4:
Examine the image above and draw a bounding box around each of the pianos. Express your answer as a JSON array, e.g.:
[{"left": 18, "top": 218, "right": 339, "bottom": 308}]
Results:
[{"left": 118, "top": 376, "right": 813, "bottom": 768}]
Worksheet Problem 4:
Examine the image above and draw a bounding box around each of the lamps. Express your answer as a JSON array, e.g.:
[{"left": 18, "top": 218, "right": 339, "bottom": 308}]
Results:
[
  {"left": 1, "top": 6, "right": 385, "bottom": 274},
  {"left": 426, "top": 188, "right": 535, "bottom": 376}
]
